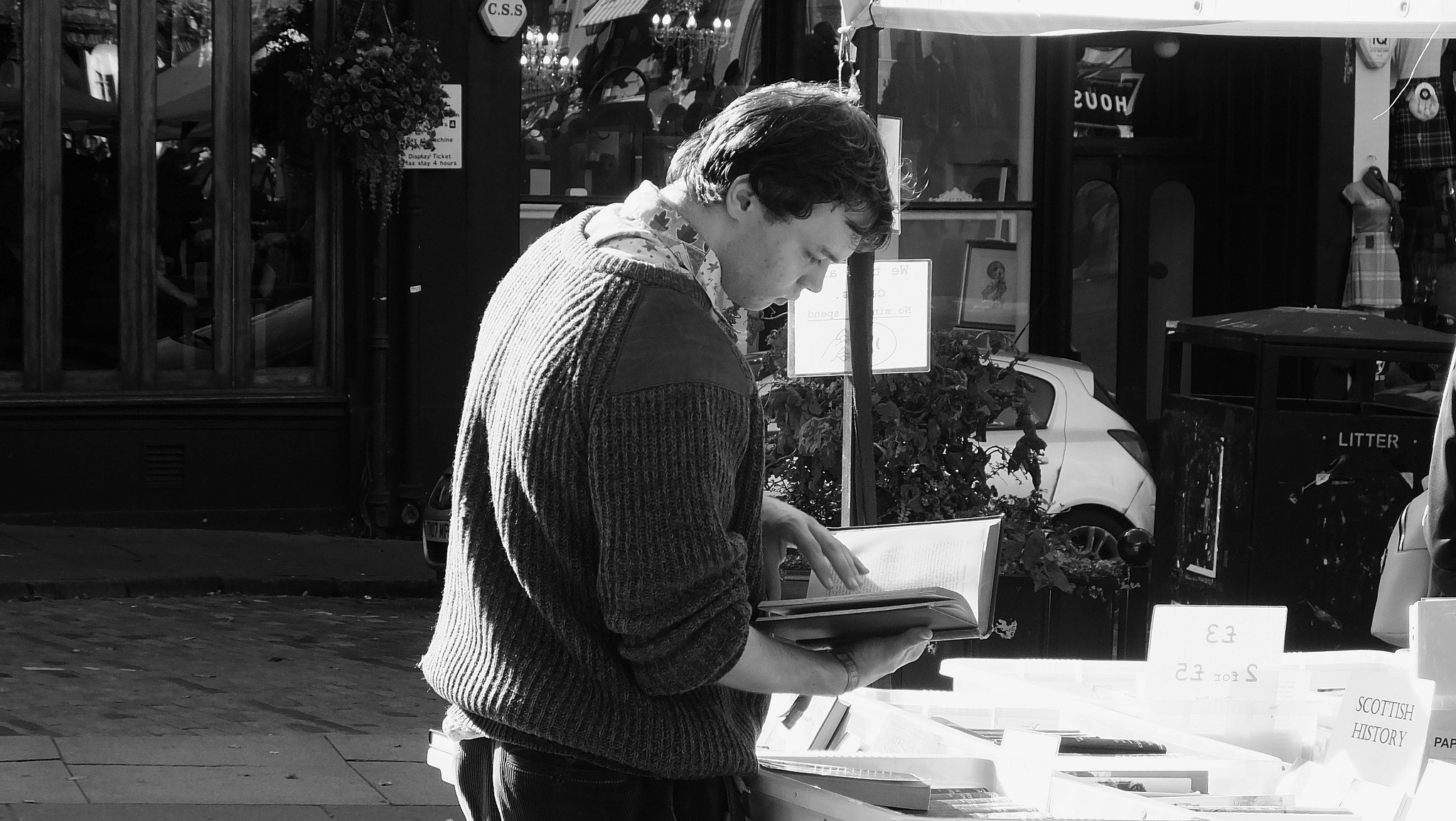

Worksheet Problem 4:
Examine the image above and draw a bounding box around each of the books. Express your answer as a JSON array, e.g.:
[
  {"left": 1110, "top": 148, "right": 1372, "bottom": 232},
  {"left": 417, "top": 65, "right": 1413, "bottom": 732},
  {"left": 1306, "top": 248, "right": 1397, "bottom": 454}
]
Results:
[{"left": 755, "top": 512, "right": 1007, "bottom": 643}]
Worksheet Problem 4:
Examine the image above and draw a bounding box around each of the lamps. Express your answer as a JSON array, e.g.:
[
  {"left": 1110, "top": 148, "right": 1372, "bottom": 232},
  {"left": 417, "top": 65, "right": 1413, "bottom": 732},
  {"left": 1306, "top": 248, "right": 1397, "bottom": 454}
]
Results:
[
  {"left": 647, "top": 0, "right": 735, "bottom": 70},
  {"left": 521, "top": 24, "right": 580, "bottom": 102}
]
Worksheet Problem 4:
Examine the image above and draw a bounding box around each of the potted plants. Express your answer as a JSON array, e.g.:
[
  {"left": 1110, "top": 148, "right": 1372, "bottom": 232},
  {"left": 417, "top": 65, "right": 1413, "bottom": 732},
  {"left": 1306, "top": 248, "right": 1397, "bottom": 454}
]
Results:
[{"left": 744, "top": 314, "right": 1136, "bottom": 659}]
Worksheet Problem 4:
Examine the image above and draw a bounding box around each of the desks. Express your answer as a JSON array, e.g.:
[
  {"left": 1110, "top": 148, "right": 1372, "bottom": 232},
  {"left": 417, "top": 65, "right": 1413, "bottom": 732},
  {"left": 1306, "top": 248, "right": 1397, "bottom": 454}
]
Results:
[{"left": 755, "top": 648, "right": 1456, "bottom": 821}]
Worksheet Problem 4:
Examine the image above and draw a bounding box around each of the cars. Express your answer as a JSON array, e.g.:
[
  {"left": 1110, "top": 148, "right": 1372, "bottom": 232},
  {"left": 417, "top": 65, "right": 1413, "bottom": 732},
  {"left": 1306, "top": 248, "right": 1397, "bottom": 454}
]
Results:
[{"left": 747, "top": 350, "right": 1159, "bottom": 593}]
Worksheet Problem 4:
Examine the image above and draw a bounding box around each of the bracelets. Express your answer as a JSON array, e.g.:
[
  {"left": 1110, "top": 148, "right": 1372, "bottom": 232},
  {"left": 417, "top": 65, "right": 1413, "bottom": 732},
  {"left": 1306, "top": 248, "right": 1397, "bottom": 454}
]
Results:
[{"left": 781, "top": 648, "right": 860, "bottom": 730}]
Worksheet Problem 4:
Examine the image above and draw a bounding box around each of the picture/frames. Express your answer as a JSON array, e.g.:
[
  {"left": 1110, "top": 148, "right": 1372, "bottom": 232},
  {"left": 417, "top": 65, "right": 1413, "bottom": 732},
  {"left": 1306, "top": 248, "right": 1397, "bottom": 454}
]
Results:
[{"left": 955, "top": 238, "right": 1019, "bottom": 329}]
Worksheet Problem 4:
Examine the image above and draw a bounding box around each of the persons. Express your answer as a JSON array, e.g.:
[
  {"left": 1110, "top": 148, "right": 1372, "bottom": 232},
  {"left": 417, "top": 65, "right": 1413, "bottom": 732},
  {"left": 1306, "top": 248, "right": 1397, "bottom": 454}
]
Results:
[{"left": 419, "top": 80, "right": 934, "bottom": 821}]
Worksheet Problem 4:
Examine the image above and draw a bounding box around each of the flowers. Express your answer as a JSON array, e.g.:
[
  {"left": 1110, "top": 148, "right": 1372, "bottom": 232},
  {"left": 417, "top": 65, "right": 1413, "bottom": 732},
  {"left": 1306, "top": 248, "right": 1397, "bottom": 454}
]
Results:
[{"left": 281, "top": 18, "right": 459, "bottom": 230}]
